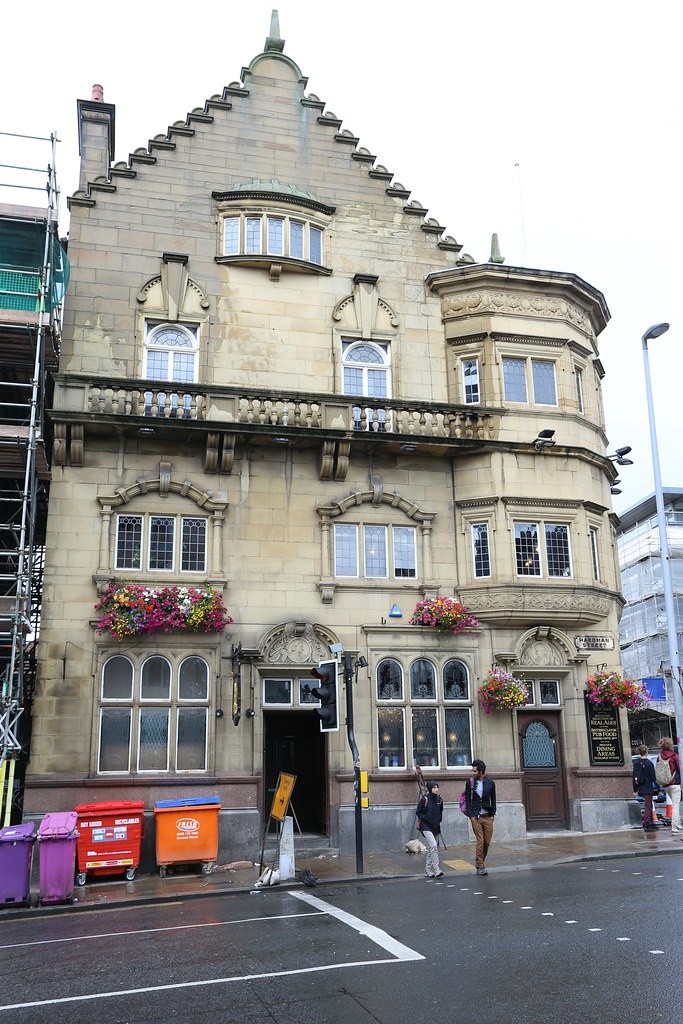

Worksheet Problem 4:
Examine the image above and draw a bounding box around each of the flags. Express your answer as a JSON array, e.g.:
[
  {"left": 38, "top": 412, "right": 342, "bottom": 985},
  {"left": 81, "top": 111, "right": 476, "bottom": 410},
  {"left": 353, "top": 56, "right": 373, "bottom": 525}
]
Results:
[{"left": 641, "top": 678, "right": 667, "bottom": 701}]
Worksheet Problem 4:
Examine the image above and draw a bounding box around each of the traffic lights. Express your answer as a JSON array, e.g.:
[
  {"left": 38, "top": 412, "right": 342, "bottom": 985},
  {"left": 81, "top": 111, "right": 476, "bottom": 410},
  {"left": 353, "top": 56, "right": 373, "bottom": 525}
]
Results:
[{"left": 311, "top": 659, "right": 340, "bottom": 732}]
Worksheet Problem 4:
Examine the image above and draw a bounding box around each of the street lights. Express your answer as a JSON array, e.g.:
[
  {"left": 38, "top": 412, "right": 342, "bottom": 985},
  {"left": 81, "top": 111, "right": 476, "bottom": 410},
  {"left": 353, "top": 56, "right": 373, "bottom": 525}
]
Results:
[{"left": 640, "top": 323, "right": 683, "bottom": 827}]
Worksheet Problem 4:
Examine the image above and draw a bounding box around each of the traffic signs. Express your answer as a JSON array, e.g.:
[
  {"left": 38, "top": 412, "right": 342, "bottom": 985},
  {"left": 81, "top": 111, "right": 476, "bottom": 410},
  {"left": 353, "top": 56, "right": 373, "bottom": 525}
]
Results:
[{"left": 270, "top": 772, "right": 297, "bottom": 823}]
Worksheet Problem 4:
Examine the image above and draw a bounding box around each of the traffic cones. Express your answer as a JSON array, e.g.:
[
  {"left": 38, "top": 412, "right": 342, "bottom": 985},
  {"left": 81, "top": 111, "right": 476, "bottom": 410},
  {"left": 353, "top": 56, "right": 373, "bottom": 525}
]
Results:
[{"left": 665, "top": 794, "right": 672, "bottom": 818}]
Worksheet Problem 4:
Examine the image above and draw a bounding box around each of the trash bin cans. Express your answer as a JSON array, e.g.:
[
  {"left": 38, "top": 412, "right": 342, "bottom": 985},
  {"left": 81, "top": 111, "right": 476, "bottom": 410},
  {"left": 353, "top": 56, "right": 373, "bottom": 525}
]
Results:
[
  {"left": 73, "top": 801, "right": 145, "bottom": 885},
  {"left": 154, "top": 796, "right": 221, "bottom": 877},
  {"left": 36, "top": 810, "right": 80, "bottom": 906},
  {"left": 0, "top": 821, "right": 38, "bottom": 907}
]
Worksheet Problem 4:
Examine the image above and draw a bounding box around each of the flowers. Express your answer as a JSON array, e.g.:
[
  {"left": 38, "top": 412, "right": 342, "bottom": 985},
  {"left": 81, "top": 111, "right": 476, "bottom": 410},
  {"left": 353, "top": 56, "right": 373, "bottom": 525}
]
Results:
[
  {"left": 474, "top": 664, "right": 535, "bottom": 713},
  {"left": 91, "top": 573, "right": 234, "bottom": 642},
  {"left": 583, "top": 667, "right": 654, "bottom": 715},
  {"left": 408, "top": 593, "right": 481, "bottom": 637}
]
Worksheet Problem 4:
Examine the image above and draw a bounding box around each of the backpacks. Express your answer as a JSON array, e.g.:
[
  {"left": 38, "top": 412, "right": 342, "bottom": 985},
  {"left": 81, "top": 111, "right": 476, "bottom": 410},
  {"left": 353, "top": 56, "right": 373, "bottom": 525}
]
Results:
[
  {"left": 459, "top": 778, "right": 474, "bottom": 815},
  {"left": 655, "top": 752, "right": 676, "bottom": 786},
  {"left": 633, "top": 760, "right": 649, "bottom": 786}
]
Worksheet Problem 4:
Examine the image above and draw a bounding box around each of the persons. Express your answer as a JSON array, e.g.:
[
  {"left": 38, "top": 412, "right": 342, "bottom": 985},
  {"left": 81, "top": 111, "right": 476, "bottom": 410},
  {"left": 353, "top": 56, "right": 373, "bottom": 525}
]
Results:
[
  {"left": 656, "top": 737, "right": 683, "bottom": 836},
  {"left": 416, "top": 780, "right": 444, "bottom": 878},
  {"left": 465, "top": 759, "right": 497, "bottom": 876},
  {"left": 632, "top": 745, "right": 662, "bottom": 832}
]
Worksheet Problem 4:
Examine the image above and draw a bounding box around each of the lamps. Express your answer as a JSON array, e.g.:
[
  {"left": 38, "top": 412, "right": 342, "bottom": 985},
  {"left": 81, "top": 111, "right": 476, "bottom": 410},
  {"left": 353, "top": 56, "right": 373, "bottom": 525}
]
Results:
[
  {"left": 354, "top": 654, "right": 369, "bottom": 683},
  {"left": 606, "top": 446, "right": 633, "bottom": 459},
  {"left": 534, "top": 429, "right": 556, "bottom": 448},
  {"left": 400, "top": 443, "right": 417, "bottom": 454},
  {"left": 272, "top": 437, "right": 289, "bottom": 445},
  {"left": 611, "top": 487, "right": 623, "bottom": 495},
  {"left": 611, "top": 457, "right": 634, "bottom": 465},
  {"left": 137, "top": 427, "right": 156, "bottom": 437}
]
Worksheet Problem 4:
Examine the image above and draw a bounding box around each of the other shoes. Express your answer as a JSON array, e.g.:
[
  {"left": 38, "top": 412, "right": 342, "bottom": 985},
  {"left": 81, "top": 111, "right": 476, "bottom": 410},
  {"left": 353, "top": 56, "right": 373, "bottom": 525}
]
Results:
[
  {"left": 477, "top": 868, "right": 488, "bottom": 875},
  {"left": 424, "top": 872, "right": 435, "bottom": 878},
  {"left": 435, "top": 870, "right": 443, "bottom": 877},
  {"left": 644, "top": 826, "right": 659, "bottom": 832}
]
